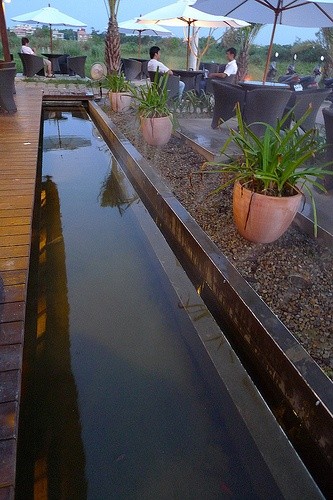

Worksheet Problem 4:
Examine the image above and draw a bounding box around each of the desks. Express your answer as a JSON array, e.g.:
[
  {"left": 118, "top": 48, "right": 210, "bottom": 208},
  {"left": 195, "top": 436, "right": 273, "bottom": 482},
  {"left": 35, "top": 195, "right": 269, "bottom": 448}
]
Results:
[
  {"left": 41, "top": 53, "right": 64, "bottom": 74},
  {"left": 239, "top": 80, "right": 292, "bottom": 92},
  {"left": 171, "top": 68, "right": 205, "bottom": 101}
]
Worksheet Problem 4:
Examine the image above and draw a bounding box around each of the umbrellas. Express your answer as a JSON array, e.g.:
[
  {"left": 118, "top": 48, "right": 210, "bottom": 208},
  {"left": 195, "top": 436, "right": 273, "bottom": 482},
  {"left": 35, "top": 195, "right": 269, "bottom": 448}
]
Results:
[
  {"left": 134, "top": 0, "right": 251, "bottom": 70},
  {"left": 9, "top": 3, "right": 87, "bottom": 53},
  {"left": 104, "top": 13, "right": 171, "bottom": 58},
  {"left": 188, "top": 0, "right": 333, "bottom": 84}
]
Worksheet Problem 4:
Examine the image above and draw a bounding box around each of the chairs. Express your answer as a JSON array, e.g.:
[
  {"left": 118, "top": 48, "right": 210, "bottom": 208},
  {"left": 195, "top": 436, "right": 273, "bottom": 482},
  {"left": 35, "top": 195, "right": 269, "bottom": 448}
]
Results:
[
  {"left": 67, "top": 55, "right": 88, "bottom": 79},
  {"left": 120, "top": 56, "right": 333, "bottom": 197},
  {"left": 0, "top": 51, "right": 46, "bottom": 114},
  {"left": 51, "top": 54, "right": 70, "bottom": 75}
]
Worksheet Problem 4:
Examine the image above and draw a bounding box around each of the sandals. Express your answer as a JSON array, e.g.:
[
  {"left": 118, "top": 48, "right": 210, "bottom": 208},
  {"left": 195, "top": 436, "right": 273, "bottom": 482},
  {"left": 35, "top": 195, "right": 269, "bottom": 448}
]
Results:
[{"left": 46, "top": 74, "right": 55, "bottom": 78}]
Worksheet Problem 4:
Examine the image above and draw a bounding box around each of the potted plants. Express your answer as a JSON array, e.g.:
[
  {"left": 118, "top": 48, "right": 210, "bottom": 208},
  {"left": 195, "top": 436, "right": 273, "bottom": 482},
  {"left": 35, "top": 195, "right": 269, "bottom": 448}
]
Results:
[
  {"left": 185, "top": 89, "right": 333, "bottom": 247},
  {"left": 86, "top": 64, "right": 140, "bottom": 113},
  {"left": 118, "top": 64, "right": 191, "bottom": 147}
]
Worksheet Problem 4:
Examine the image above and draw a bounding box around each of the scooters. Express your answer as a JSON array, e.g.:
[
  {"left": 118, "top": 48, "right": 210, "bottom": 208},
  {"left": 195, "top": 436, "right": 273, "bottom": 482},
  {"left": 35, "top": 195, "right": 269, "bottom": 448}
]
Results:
[{"left": 268, "top": 52, "right": 333, "bottom": 99}]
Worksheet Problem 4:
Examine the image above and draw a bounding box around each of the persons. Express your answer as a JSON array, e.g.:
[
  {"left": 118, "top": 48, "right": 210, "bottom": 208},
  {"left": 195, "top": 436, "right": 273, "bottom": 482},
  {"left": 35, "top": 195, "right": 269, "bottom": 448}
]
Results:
[
  {"left": 20, "top": 37, "right": 56, "bottom": 78},
  {"left": 208, "top": 47, "right": 239, "bottom": 79},
  {"left": 147, "top": 46, "right": 185, "bottom": 100}
]
